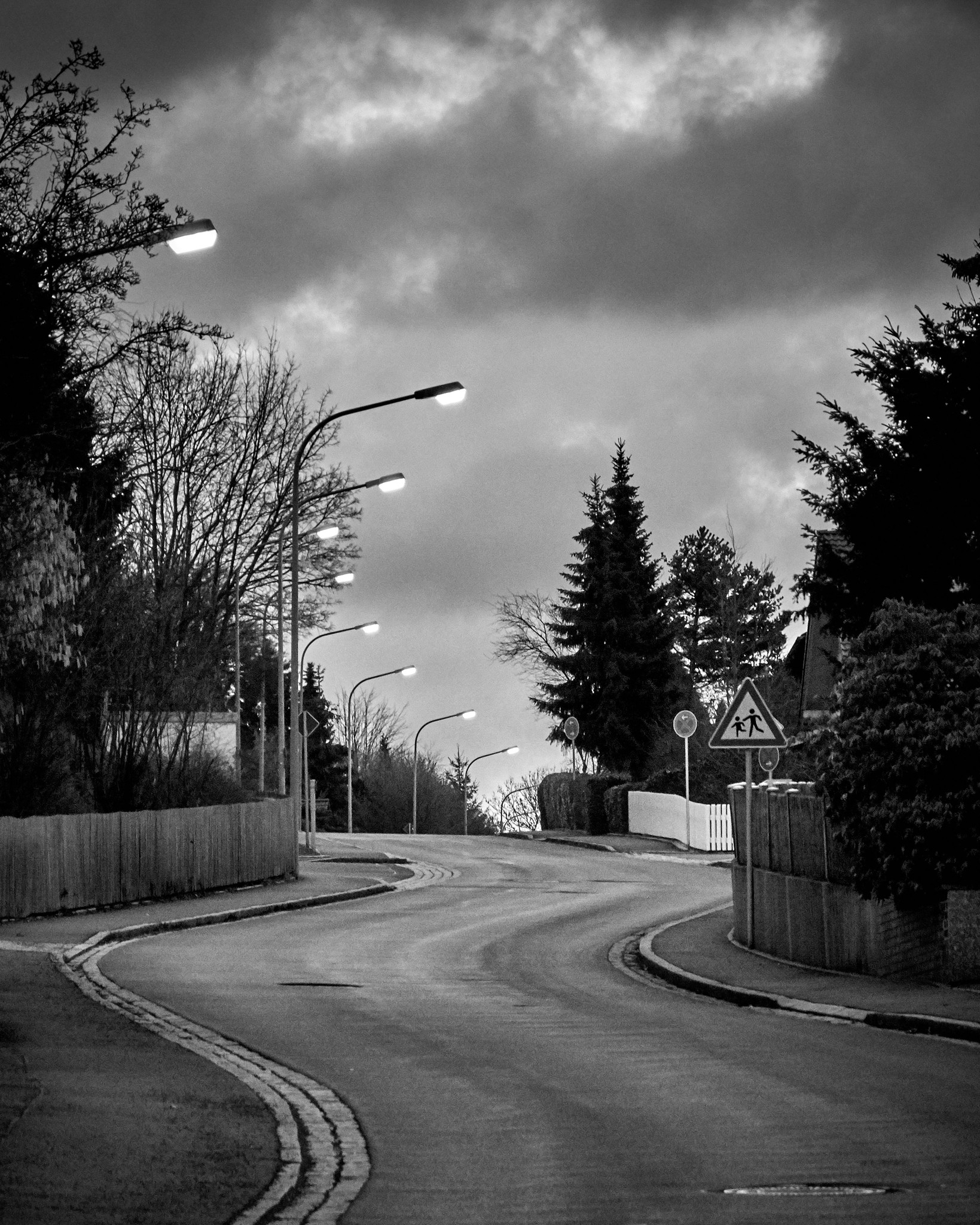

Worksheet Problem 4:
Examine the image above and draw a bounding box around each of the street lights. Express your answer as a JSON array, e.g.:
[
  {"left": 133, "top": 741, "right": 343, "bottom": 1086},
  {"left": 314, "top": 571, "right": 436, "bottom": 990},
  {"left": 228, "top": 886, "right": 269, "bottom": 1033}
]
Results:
[
  {"left": 345, "top": 664, "right": 417, "bottom": 836},
  {"left": 275, "top": 470, "right": 405, "bottom": 799},
  {"left": 464, "top": 743, "right": 520, "bottom": 836},
  {"left": 257, "top": 570, "right": 356, "bottom": 795},
  {"left": 289, "top": 381, "right": 470, "bottom": 830},
  {"left": 298, "top": 620, "right": 381, "bottom": 736},
  {"left": 234, "top": 521, "right": 341, "bottom": 796},
  {"left": 412, "top": 710, "right": 476, "bottom": 837}
]
